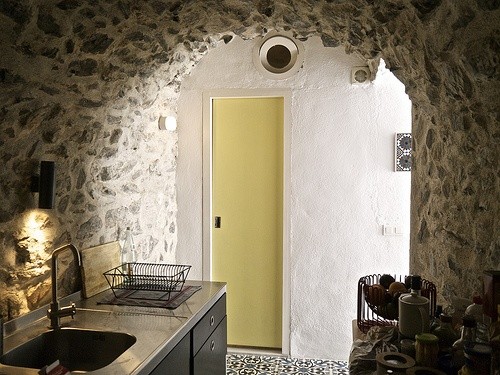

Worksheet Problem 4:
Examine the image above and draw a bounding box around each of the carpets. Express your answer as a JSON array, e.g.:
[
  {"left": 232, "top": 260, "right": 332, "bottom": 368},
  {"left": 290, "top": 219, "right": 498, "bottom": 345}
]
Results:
[{"left": 226, "top": 352, "right": 350, "bottom": 375}]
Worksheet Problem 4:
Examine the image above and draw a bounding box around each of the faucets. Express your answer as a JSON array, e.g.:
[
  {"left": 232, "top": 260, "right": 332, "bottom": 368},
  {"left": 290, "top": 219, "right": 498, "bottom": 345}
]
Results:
[{"left": 47, "top": 244, "right": 81, "bottom": 329}]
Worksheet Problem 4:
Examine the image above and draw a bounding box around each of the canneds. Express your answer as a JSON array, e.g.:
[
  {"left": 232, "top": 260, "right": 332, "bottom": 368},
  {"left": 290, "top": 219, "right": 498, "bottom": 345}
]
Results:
[
  {"left": 415, "top": 333, "right": 439, "bottom": 368},
  {"left": 463, "top": 342, "right": 492, "bottom": 375}
]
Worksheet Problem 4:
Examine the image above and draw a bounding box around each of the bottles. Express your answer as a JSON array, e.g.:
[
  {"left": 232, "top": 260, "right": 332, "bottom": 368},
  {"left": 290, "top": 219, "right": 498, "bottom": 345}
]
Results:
[
  {"left": 376, "top": 351, "right": 446, "bottom": 375},
  {"left": 411, "top": 276, "right": 421, "bottom": 296},
  {"left": 414, "top": 296, "right": 495, "bottom": 375},
  {"left": 122, "top": 227, "right": 138, "bottom": 286}
]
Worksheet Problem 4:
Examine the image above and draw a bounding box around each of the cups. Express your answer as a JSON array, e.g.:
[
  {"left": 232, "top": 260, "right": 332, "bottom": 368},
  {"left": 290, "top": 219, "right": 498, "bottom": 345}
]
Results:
[
  {"left": 398, "top": 294, "right": 431, "bottom": 338},
  {"left": 399, "top": 340, "right": 415, "bottom": 358}
]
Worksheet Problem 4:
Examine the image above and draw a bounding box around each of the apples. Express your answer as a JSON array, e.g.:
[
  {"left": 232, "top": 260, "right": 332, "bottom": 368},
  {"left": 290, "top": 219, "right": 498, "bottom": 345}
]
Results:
[{"left": 369, "top": 275, "right": 412, "bottom": 316}]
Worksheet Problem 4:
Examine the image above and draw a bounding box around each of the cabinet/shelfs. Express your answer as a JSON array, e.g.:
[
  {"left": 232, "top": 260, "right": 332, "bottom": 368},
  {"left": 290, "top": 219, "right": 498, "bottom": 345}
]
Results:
[{"left": 149, "top": 292, "right": 228, "bottom": 375}]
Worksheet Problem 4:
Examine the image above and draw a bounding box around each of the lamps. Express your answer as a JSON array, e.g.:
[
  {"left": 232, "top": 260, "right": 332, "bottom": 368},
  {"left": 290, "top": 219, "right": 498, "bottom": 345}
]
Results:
[{"left": 31, "top": 160, "right": 56, "bottom": 209}]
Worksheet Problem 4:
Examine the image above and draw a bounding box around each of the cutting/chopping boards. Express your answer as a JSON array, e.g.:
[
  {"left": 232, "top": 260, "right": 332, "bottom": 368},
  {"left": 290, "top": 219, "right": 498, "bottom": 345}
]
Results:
[{"left": 80, "top": 241, "right": 124, "bottom": 298}]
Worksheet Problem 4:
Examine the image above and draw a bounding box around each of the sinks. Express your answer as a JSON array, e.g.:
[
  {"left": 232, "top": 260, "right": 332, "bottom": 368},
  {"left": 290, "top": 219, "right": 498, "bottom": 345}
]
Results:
[{"left": 0, "top": 328, "right": 137, "bottom": 371}]
